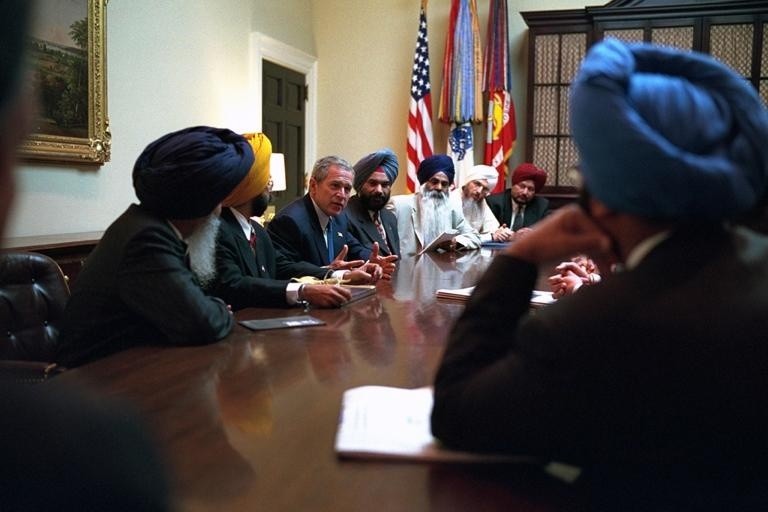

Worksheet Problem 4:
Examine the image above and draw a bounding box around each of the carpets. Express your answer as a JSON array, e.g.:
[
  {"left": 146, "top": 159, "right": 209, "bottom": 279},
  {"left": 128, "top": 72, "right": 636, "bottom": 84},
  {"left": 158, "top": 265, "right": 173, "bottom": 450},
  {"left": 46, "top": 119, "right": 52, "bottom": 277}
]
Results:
[
  {"left": 249, "top": 232, "right": 258, "bottom": 259},
  {"left": 512, "top": 203, "right": 524, "bottom": 232},
  {"left": 327, "top": 218, "right": 335, "bottom": 264},
  {"left": 373, "top": 210, "right": 391, "bottom": 253}
]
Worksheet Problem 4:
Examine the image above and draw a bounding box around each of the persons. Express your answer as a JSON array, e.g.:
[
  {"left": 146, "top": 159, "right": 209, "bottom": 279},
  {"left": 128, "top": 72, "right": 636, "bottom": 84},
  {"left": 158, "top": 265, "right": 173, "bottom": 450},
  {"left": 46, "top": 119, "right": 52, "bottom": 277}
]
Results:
[
  {"left": 385, "top": 154, "right": 481, "bottom": 255},
  {"left": 486, "top": 163, "right": 551, "bottom": 232},
  {"left": 267, "top": 155, "right": 398, "bottom": 281},
  {"left": 206, "top": 132, "right": 383, "bottom": 309},
  {"left": 451, "top": 164, "right": 512, "bottom": 242},
  {"left": 430, "top": 36, "right": 768, "bottom": 512},
  {"left": 56, "top": 126, "right": 256, "bottom": 368},
  {"left": 332, "top": 148, "right": 401, "bottom": 261},
  {"left": 1, "top": 0, "right": 174, "bottom": 512}
]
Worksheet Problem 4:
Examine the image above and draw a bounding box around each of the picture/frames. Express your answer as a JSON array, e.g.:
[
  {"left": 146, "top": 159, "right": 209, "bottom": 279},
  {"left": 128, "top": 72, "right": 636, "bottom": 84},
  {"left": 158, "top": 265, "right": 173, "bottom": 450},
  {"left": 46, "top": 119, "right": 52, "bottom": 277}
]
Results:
[{"left": 50, "top": 226, "right": 611, "bottom": 512}]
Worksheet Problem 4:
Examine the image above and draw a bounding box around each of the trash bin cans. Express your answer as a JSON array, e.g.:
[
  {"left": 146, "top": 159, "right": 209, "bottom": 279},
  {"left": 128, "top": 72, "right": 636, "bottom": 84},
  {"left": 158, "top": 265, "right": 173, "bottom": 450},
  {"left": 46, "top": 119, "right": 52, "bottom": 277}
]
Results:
[{"left": 296, "top": 284, "right": 309, "bottom": 308}]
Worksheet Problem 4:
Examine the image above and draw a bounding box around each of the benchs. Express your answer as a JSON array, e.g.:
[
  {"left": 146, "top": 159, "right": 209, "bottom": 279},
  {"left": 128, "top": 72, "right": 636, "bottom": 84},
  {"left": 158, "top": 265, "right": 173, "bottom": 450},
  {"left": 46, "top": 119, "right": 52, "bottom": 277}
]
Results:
[{"left": 406, "top": 0, "right": 434, "bottom": 193}]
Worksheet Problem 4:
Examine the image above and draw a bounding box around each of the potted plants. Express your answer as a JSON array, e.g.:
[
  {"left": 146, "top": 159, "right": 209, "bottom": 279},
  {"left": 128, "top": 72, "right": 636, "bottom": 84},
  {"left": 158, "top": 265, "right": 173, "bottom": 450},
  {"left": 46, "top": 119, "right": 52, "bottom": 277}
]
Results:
[{"left": 0, "top": 0, "right": 114, "bottom": 168}]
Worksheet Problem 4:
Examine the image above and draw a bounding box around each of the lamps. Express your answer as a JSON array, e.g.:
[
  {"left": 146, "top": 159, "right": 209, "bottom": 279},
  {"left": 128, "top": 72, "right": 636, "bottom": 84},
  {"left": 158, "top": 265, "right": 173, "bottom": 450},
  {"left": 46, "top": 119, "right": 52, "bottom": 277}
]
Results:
[{"left": 2, "top": 251, "right": 75, "bottom": 384}]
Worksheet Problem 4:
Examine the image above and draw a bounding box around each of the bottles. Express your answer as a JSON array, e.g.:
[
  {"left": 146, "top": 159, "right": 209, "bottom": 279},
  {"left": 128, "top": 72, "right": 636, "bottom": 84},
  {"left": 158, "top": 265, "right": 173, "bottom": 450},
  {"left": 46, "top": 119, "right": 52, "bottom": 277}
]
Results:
[
  {"left": 511, "top": 163, "right": 547, "bottom": 193},
  {"left": 416, "top": 154, "right": 456, "bottom": 187},
  {"left": 353, "top": 147, "right": 400, "bottom": 192},
  {"left": 132, "top": 125, "right": 256, "bottom": 220},
  {"left": 461, "top": 164, "right": 500, "bottom": 193},
  {"left": 220, "top": 131, "right": 273, "bottom": 208},
  {"left": 566, "top": 37, "right": 768, "bottom": 226}
]
